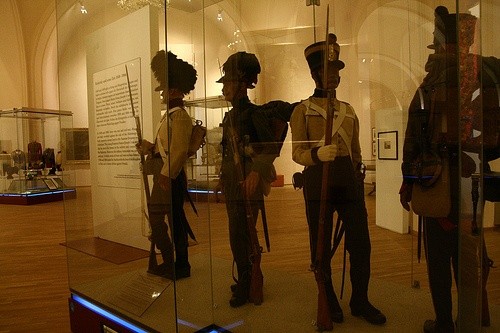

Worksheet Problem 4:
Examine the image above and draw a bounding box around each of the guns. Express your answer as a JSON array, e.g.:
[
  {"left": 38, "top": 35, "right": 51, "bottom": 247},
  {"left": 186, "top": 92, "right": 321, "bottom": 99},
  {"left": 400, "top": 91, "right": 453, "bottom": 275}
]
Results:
[
  {"left": 217, "top": 59, "right": 264, "bottom": 305},
  {"left": 125, "top": 63, "right": 158, "bottom": 273},
  {"left": 317, "top": 3, "right": 335, "bottom": 329}
]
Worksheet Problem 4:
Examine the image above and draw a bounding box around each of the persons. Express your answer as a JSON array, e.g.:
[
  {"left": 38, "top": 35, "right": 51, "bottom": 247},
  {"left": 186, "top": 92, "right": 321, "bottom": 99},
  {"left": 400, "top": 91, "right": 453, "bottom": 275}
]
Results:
[
  {"left": 131, "top": 50, "right": 197, "bottom": 279},
  {"left": 12, "top": 149, "right": 27, "bottom": 170},
  {"left": 55, "top": 152, "right": 63, "bottom": 166},
  {"left": 210, "top": 50, "right": 278, "bottom": 307},
  {"left": 399, "top": 12, "right": 490, "bottom": 333},
  {"left": 28, "top": 140, "right": 43, "bottom": 163},
  {"left": 42, "top": 146, "right": 56, "bottom": 174},
  {"left": 291, "top": 41, "right": 387, "bottom": 324}
]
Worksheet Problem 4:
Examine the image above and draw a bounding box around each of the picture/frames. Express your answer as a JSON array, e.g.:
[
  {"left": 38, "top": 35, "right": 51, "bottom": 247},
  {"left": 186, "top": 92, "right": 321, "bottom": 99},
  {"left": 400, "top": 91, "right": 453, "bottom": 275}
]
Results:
[{"left": 378, "top": 130, "right": 398, "bottom": 160}]
